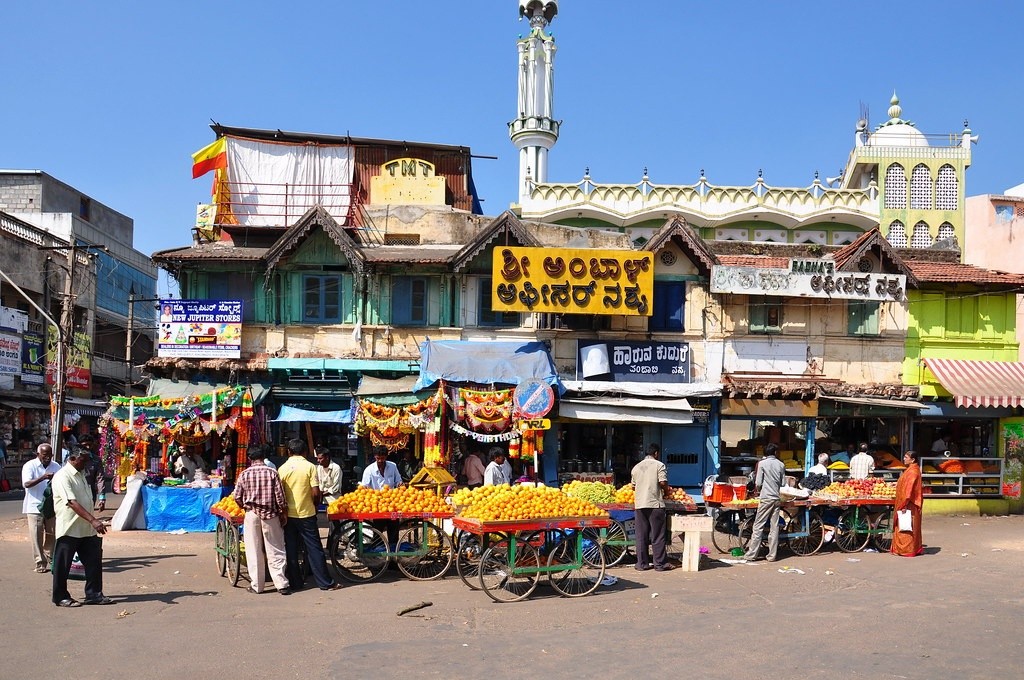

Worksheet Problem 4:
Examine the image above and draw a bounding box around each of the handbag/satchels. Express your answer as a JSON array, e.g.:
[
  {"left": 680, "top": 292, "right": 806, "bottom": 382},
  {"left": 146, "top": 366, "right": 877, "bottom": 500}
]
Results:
[
  {"left": 37, "top": 481, "right": 55, "bottom": 519},
  {"left": 896, "top": 510, "right": 914, "bottom": 532}
]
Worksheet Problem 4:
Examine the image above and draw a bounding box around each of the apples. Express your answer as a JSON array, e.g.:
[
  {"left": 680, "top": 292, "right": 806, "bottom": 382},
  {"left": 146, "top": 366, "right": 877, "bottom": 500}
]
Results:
[
  {"left": 663, "top": 486, "right": 691, "bottom": 503},
  {"left": 819, "top": 477, "right": 898, "bottom": 498}
]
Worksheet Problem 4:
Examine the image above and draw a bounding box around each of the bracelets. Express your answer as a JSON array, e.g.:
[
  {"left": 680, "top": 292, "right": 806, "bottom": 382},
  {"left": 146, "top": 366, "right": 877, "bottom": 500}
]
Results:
[{"left": 100, "top": 501, "right": 105, "bottom": 504}]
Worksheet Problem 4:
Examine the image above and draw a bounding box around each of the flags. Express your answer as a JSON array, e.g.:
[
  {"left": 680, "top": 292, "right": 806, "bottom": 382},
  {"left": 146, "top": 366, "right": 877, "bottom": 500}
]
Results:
[{"left": 191, "top": 136, "right": 227, "bottom": 180}]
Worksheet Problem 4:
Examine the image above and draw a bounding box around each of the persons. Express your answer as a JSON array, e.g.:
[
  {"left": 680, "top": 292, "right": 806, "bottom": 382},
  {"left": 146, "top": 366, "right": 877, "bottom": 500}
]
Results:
[
  {"left": 631, "top": 442, "right": 676, "bottom": 571},
  {"left": 0, "top": 430, "right": 8, "bottom": 492},
  {"left": 740, "top": 443, "right": 786, "bottom": 561},
  {"left": 931, "top": 434, "right": 951, "bottom": 467},
  {"left": 891, "top": 450, "right": 924, "bottom": 557},
  {"left": 46, "top": 429, "right": 106, "bottom": 562},
  {"left": 51, "top": 443, "right": 114, "bottom": 607},
  {"left": 235, "top": 445, "right": 290, "bottom": 595},
  {"left": 277, "top": 438, "right": 344, "bottom": 592},
  {"left": 806, "top": 441, "right": 875, "bottom": 479},
  {"left": 457, "top": 443, "right": 513, "bottom": 491},
  {"left": 357, "top": 444, "right": 406, "bottom": 544},
  {"left": 173, "top": 443, "right": 205, "bottom": 481},
  {"left": 161, "top": 304, "right": 172, "bottom": 322},
  {"left": 22, "top": 443, "right": 62, "bottom": 573}
]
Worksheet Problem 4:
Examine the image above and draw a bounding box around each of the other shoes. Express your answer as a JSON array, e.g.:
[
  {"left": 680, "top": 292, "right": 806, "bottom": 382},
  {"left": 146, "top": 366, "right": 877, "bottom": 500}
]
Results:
[
  {"left": 37, "top": 561, "right": 46, "bottom": 572},
  {"left": 279, "top": 586, "right": 290, "bottom": 594},
  {"left": 327, "top": 583, "right": 343, "bottom": 591},
  {"left": 245, "top": 585, "right": 265, "bottom": 594},
  {"left": 636, "top": 565, "right": 652, "bottom": 571},
  {"left": 658, "top": 563, "right": 675, "bottom": 571},
  {"left": 293, "top": 582, "right": 308, "bottom": 589},
  {"left": 46, "top": 554, "right": 52, "bottom": 564}
]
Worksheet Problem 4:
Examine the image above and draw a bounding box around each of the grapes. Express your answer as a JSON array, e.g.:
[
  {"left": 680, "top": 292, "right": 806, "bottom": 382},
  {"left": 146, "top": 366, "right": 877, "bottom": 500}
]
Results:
[{"left": 568, "top": 482, "right": 616, "bottom": 504}]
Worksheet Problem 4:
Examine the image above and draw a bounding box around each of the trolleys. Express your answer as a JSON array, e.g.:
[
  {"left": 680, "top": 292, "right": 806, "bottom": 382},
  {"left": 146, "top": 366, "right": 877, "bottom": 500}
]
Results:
[
  {"left": 206, "top": 493, "right": 900, "bottom": 589},
  {"left": 448, "top": 514, "right": 614, "bottom": 603}
]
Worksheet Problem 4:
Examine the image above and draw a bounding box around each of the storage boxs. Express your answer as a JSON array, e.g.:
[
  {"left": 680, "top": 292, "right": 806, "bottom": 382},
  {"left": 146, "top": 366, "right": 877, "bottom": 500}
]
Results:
[{"left": 703, "top": 485, "right": 748, "bottom": 503}]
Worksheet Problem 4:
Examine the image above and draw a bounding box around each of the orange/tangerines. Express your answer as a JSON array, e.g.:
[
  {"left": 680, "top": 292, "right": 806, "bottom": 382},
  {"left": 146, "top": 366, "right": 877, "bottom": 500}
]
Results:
[
  {"left": 212, "top": 493, "right": 245, "bottom": 517},
  {"left": 452, "top": 480, "right": 606, "bottom": 524},
  {"left": 614, "top": 483, "right": 634, "bottom": 503},
  {"left": 327, "top": 484, "right": 455, "bottom": 514}
]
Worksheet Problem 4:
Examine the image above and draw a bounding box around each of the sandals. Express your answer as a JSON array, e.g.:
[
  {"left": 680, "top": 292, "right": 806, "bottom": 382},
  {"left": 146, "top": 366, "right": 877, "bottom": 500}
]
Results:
[
  {"left": 84, "top": 597, "right": 112, "bottom": 604},
  {"left": 56, "top": 598, "right": 80, "bottom": 607}
]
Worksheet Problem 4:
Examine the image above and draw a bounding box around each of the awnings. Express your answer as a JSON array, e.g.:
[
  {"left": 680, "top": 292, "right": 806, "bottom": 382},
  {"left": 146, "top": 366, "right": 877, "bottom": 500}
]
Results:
[
  {"left": 818, "top": 394, "right": 930, "bottom": 410},
  {"left": 923, "top": 357, "right": 1024, "bottom": 408},
  {"left": 64, "top": 396, "right": 111, "bottom": 416},
  {"left": 266, "top": 404, "right": 354, "bottom": 423}
]
